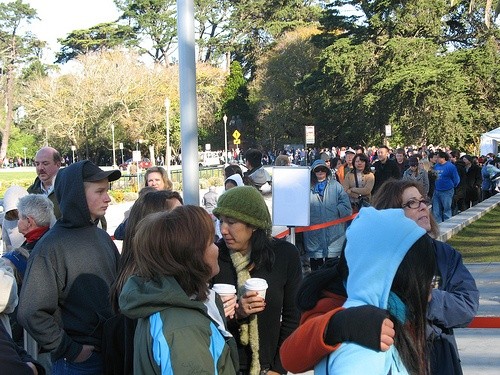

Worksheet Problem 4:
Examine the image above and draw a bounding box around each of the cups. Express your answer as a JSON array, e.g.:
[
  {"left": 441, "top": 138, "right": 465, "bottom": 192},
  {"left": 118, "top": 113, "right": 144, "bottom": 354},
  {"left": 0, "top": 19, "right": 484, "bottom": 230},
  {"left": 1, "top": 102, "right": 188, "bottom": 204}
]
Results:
[
  {"left": 244, "top": 278, "right": 269, "bottom": 302},
  {"left": 211, "top": 283, "right": 237, "bottom": 307},
  {"left": 371, "top": 167, "right": 375, "bottom": 175}
]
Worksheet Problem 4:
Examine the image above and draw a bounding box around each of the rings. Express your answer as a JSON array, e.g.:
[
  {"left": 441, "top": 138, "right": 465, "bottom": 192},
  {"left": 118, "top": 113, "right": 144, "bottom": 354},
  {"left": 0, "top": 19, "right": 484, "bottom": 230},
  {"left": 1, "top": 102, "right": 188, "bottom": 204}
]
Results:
[
  {"left": 223, "top": 303, "right": 226, "bottom": 308},
  {"left": 249, "top": 304, "right": 252, "bottom": 310}
]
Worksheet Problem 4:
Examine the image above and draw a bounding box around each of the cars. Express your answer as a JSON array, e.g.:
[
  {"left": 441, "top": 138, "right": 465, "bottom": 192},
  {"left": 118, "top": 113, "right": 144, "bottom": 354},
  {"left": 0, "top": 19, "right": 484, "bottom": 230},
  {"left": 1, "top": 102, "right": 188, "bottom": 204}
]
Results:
[
  {"left": 118, "top": 157, "right": 152, "bottom": 171},
  {"left": 199, "top": 152, "right": 221, "bottom": 167}
]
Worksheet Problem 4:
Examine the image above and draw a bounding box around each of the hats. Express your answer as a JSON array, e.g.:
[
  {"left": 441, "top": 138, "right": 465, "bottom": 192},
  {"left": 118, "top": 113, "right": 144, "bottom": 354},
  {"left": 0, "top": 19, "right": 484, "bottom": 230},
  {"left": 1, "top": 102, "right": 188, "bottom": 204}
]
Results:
[
  {"left": 212, "top": 185, "right": 272, "bottom": 243},
  {"left": 82, "top": 162, "right": 121, "bottom": 182},
  {"left": 408, "top": 158, "right": 418, "bottom": 165},
  {"left": 346, "top": 149, "right": 355, "bottom": 153}
]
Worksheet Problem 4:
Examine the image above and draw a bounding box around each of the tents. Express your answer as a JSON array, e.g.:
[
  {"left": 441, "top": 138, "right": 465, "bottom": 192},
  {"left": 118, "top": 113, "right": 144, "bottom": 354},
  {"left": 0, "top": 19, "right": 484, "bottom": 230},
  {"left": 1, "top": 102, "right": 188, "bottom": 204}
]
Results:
[{"left": 480, "top": 127, "right": 500, "bottom": 156}]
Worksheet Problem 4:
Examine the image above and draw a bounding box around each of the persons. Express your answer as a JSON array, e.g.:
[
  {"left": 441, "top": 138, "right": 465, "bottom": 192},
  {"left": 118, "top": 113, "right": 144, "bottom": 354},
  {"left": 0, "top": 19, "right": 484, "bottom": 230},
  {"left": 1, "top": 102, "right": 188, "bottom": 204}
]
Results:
[{"left": 0, "top": 144, "right": 500, "bottom": 375}]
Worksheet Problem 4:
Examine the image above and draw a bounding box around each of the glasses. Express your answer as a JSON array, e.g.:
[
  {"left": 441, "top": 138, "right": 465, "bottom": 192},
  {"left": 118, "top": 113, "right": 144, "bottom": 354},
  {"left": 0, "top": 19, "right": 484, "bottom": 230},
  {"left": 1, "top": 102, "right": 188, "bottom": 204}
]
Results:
[
  {"left": 404, "top": 199, "right": 432, "bottom": 209},
  {"left": 315, "top": 167, "right": 327, "bottom": 172}
]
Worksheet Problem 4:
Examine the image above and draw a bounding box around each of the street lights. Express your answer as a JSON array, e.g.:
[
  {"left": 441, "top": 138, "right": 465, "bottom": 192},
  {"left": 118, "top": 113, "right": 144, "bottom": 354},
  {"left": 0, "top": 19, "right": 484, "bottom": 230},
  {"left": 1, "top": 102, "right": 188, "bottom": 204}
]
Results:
[
  {"left": 110, "top": 123, "right": 115, "bottom": 169},
  {"left": 222, "top": 114, "right": 227, "bottom": 167},
  {"left": 163, "top": 97, "right": 171, "bottom": 175}
]
